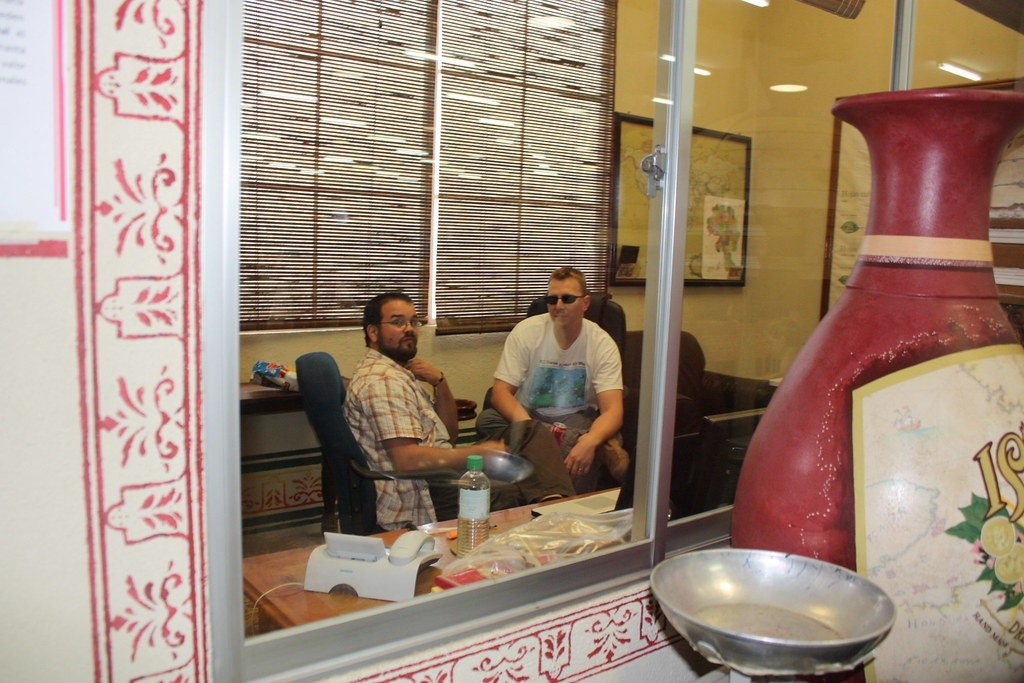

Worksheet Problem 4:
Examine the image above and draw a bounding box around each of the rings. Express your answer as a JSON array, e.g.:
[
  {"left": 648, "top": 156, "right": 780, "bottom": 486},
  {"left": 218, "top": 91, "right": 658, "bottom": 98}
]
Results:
[{"left": 578, "top": 470, "right": 583, "bottom": 472}]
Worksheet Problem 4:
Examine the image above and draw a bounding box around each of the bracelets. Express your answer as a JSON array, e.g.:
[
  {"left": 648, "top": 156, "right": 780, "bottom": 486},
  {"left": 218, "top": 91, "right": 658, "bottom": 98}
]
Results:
[{"left": 434, "top": 372, "right": 444, "bottom": 387}]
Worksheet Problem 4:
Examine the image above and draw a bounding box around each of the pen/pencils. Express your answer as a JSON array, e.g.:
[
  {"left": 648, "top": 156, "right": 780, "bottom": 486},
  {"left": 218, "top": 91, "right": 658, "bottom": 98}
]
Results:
[{"left": 425, "top": 524, "right": 498, "bottom": 534}]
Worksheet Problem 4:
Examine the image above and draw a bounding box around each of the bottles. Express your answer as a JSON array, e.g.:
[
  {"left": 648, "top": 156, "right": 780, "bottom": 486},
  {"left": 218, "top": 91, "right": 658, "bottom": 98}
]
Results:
[{"left": 457, "top": 456, "right": 491, "bottom": 559}]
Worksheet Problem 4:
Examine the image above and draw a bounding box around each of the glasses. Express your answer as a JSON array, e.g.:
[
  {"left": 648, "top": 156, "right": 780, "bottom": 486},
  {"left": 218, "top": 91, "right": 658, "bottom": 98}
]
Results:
[
  {"left": 546, "top": 294, "right": 584, "bottom": 305},
  {"left": 377, "top": 320, "right": 422, "bottom": 328}
]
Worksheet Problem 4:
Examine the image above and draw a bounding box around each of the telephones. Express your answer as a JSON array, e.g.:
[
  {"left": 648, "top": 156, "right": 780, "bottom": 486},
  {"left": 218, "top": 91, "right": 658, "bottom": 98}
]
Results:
[{"left": 304, "top": 530, "right": 443, "bottom": 602}]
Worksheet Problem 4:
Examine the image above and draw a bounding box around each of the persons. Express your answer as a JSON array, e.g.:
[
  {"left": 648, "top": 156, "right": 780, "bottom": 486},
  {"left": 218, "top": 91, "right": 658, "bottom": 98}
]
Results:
[
  {"left": 341, "top": 293, "right": 575, "bottom": 532},
  {"left": 475, "top": 265, "right": 630, "bottom": 495}
]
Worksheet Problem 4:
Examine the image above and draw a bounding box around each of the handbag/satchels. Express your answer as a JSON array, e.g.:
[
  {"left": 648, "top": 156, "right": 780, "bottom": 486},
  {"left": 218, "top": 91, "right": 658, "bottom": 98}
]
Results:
[{"left": 615, "top": 410, "right": 730, "bottom": 520}]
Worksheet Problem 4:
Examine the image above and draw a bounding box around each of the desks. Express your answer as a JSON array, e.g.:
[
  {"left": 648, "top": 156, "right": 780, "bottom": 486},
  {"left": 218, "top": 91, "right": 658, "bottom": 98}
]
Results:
[
  {"left": 242, "top": 488, "right": 635, "bottom": 630},
  {"left": 239, "top": 375, "right": 353, "bottom": 537}
]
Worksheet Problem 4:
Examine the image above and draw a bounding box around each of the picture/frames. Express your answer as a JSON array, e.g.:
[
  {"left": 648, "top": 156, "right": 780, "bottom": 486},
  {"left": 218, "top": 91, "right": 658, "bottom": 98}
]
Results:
[{"left": 609, "top": 111, "right": 752, "bottom": 288}]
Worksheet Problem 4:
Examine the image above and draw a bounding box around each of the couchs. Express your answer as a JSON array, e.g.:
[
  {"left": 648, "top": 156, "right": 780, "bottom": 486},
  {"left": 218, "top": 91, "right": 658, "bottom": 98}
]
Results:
[{"left": 617, "top": 329, "right": 769, "bottom": 524}]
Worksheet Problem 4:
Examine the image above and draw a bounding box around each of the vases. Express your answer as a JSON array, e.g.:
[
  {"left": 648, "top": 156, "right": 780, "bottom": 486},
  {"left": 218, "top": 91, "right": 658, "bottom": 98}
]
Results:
[{"left": 731, "top": 88, "right": 1024, "bottom": 683}]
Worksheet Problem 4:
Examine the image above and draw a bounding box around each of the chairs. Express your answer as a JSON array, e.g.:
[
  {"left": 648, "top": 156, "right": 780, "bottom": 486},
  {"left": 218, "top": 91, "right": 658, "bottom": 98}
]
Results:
[
  {"left": 526, "top": 294, "right": 625, "bottom": 353},
  {"left": 296, "top": 351, "right": 462, "bottom": 537}
]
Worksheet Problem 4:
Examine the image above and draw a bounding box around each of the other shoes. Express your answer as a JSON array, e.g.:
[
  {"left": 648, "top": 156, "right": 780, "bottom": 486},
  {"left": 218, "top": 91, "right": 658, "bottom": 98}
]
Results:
[{"left": 539, "top": 494, "right": 562, "bottom": 502}]
